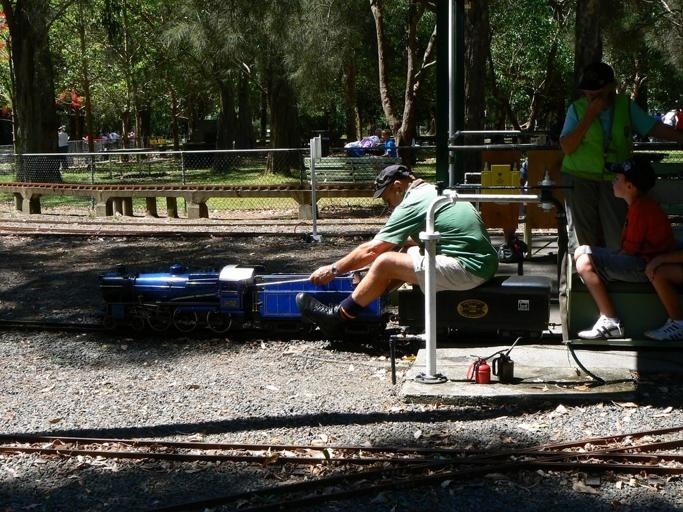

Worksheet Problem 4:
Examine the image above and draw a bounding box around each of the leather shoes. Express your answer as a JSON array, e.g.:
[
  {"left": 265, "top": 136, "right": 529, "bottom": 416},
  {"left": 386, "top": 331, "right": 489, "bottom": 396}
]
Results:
[{"left": 296, "top": 293, "right": 343, "bottom": 329}]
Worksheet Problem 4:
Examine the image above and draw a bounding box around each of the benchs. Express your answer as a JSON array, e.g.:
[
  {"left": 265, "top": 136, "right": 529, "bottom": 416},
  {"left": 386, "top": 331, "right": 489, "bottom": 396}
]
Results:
[{"left": 305, "top": 156, "right": 404, "bottom": 188}]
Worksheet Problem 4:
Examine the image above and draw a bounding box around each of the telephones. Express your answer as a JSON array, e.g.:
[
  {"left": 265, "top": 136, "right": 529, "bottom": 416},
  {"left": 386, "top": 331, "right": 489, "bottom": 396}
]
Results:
[{"left": 498, "top": 240, "right": 529, "bottom": 264}]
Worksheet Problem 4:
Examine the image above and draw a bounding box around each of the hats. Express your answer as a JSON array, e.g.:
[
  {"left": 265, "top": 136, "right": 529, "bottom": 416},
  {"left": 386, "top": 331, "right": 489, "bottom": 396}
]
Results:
[
  {"left": 373, "top": 164, "right": 410, "bottom": 198},
  {"left": 576, "top": 63, "right": 614, "bottom": 90},
  {"left": 606, "top": 158, "right": 657, "bottom": 187}
]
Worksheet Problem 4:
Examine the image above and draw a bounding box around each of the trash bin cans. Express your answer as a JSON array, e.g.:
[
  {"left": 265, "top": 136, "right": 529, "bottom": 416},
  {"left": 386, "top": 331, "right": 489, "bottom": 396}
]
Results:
[
  {"left": 310, "top": 133, "right": 329, "bottom": 158},
  {"left": 448, "top": 130, "right": 533, "bottom": 185},
  {"left": 182, "top": 143, "right": 201, "bottom": 168}
]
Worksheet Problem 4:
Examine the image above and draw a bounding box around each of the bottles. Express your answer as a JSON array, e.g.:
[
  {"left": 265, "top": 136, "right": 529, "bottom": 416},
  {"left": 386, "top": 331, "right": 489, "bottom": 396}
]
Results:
[
  {"left": 476, "top": 360, "right": 491, "bottom": 384},
  {"left": 541, "top": 170, "right": 554, "bottom": 210},
  {"left": 500, "top": 356, "right": 514, "bottom": 385}
]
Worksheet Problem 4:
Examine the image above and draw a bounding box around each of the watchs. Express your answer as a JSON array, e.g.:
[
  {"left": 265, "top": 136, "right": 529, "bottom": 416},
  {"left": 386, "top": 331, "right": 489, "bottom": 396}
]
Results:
[{"left": 330, "top": 263, "right": 341, "bottom": 278}]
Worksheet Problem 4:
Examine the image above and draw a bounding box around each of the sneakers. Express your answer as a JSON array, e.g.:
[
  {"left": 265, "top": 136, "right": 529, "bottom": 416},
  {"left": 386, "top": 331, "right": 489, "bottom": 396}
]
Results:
[
  {"left": 645, "top": 319, "right": 683, "bottom": 340},
  {"left": 578, "top": 315, "right": 625, "bottom": 339}
]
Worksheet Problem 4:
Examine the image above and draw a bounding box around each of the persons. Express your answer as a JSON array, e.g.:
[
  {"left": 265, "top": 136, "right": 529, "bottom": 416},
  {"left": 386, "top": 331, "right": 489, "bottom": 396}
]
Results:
[
  {"left": 643, "top": 252, "right": 682, "bottom": 344},
  {"left": 558, "top": 64, "right": 682, "bottom": 254},
  {"left": 520, "top": 158, "right": 529, "bottom": 189},
  {"left": 81, "top": 128, "right": 135, "bottom": 154},
  {"left": 56, "top": 126, "right": 70, "bottom": 170},
  {"left": 654, "top": 109, "right": 682, "bottom": 131},
  {"left": 572, "top": 156, "right": 673, "bottom": 341},
  {"left": 294, "top": 165, "right": 498, "bottom": 340},
  {"left": 377, "top": 129, "right": 396, "bottom": 165}
]
